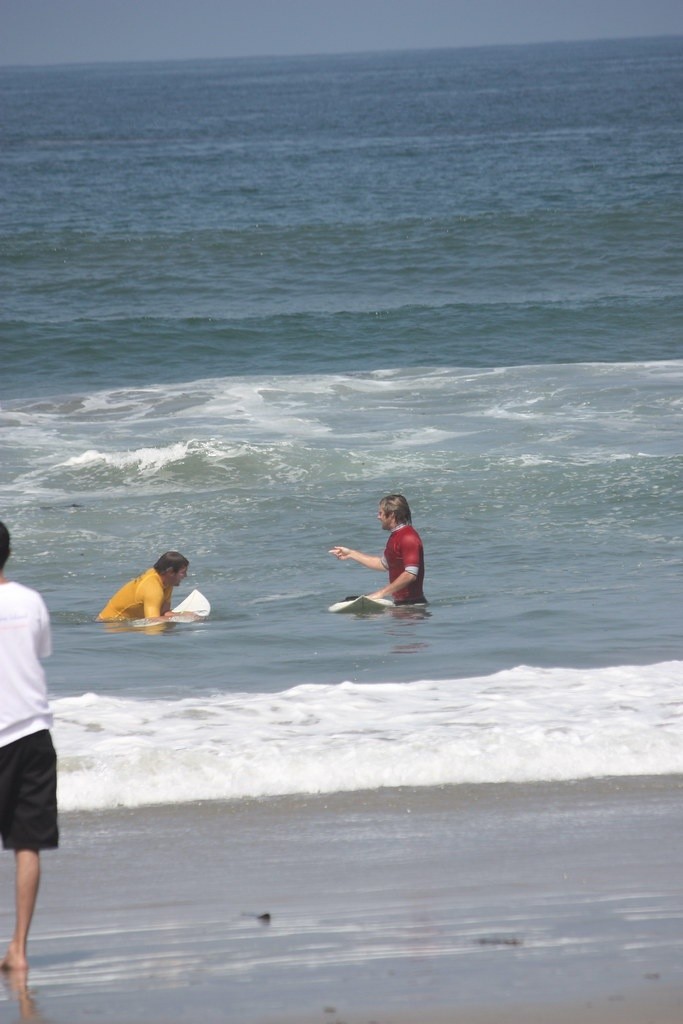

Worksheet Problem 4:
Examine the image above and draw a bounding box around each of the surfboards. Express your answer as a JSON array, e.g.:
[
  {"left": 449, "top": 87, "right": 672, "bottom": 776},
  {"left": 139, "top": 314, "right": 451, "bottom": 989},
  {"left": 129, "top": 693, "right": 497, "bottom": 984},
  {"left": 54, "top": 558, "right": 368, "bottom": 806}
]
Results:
[
  {"left": 328, "top": 595, "right": 395, "bottom": 614},
  {"left": 173, "top": 588, "right": 211, "bottom": 618}
]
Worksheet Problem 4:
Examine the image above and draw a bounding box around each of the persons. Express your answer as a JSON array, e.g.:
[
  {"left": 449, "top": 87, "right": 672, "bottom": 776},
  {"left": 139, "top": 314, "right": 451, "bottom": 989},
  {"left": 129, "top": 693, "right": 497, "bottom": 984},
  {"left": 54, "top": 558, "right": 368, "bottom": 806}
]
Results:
[
  {"left": 0, "top": 519, "right": 62, "bottom": 972},
  {"left": 328, "top": 494, "right": 428, "bottom": 606},
  {"left": 95, "top": 549, "right": 210, "bottom": 635}
]
